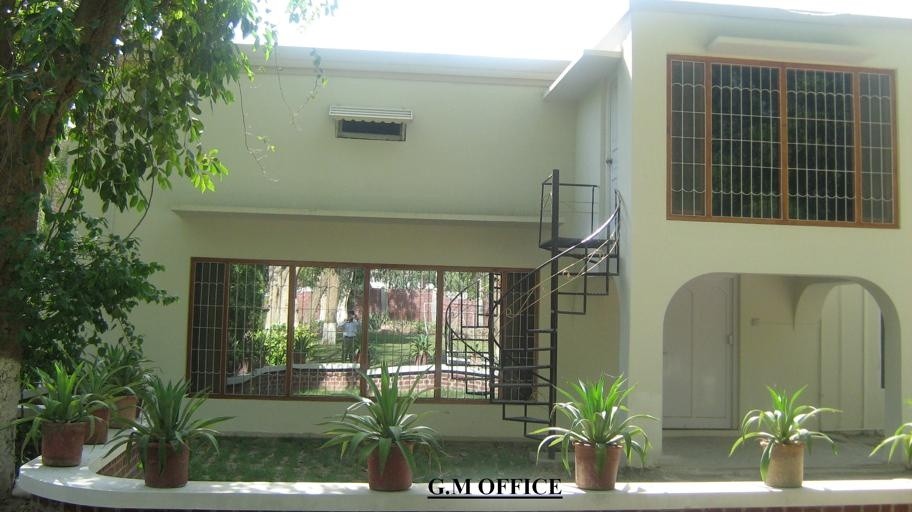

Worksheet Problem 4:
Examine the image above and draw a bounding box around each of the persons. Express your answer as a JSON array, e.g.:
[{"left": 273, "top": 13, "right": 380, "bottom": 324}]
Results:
[{"left": 336, "top": 310, "right": 361, "bottom": 364}]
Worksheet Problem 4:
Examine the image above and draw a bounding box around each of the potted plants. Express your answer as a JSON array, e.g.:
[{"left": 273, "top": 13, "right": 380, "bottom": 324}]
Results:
[
  {"left": 350, "top": 328, "right": 375, "bottom": 364},
  {"left": 526, "top": 370, "right": 661, "bottom": 491},
  {"left": 407, "top": 332, "right": 433, "bottom": 365},
  {"left": 233, "top": 330, "right": 274, "bottom": 376},
  {"left": 12, "top": 342, "right": 235, "bottom": 488},
  {"left": 729, "top": 387, "right": 844, "bottom": 489},
  {"left": 315, "top": 362, "right": 451, "bottom": 492},
  {"left": 293, "top": 329, "right": 314, "bottom": 364}
]
